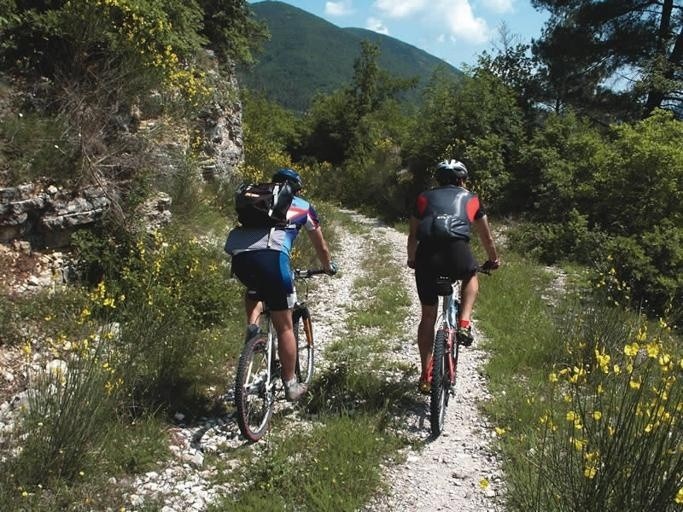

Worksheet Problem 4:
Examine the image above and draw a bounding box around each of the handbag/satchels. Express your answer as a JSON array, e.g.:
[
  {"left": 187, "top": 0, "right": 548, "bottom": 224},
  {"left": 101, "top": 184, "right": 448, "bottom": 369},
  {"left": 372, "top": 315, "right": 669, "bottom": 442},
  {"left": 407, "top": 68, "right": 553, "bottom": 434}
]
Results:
[{"left": 416, "top": 214, "right": 469, "bottom": 239}]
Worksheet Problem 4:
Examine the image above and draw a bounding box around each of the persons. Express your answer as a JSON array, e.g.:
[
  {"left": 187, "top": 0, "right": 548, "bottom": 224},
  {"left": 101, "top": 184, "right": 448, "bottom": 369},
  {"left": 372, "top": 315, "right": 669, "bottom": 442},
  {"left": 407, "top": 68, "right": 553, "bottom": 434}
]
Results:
[
  {"left": 230, "top": 166, "right": 336, "bottom": 401},
  {"left": 407, "top": 156, "right": 500, "bottom": 393}
]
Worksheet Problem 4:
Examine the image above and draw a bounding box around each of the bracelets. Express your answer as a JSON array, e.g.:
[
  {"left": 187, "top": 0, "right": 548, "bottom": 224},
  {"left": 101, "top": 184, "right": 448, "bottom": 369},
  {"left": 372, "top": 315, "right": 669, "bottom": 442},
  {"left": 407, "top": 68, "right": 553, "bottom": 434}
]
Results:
[{"left": 489, "top": 258, "right": 498, "bottom": 263}]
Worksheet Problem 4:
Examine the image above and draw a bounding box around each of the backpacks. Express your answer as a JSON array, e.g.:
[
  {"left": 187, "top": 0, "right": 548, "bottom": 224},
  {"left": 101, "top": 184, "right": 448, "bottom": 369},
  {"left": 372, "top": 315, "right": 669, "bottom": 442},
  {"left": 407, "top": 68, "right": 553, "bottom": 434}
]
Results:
[{"left": 236, "top": 180, "right": 293, "bottom": 227}]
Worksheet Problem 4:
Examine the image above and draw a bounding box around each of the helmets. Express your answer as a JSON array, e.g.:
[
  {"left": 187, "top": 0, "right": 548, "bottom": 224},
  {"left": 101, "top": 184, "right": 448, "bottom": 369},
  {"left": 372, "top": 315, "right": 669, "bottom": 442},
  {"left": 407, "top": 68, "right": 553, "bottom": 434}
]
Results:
[
  {"left": 435, "top": 159, "right": 468, "bottom": 177},
  {"left": 272, "top": 167, "right": 303, "bottom": 190}
]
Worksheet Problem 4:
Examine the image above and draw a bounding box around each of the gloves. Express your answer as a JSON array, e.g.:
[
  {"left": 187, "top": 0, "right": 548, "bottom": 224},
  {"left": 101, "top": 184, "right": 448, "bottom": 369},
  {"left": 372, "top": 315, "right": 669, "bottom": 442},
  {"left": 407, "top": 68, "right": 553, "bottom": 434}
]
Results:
[
  {"left": 321, "top": 261, "right": 337, "bottom": 275},
  {"left": 484, "top": 258, "right": 499, "bottom": 269},
  {"left": 408, "top": 259, "right": 416, "bottom": 269}
]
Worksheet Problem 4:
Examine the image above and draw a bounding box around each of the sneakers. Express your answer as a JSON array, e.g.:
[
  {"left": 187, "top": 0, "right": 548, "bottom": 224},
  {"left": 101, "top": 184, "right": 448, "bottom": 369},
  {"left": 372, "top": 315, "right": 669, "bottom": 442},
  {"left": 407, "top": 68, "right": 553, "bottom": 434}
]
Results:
[
  {"left": 457, "top": 327, "right": 474, "bottom": 346},
  {"left": 285, "top": 382, "right": 307, "bottom": 401},
  {"left": 245, "top": 323, "right": 262, "bottom": 352},
  {"left": 419, "top": 378, "right": 431, "bottom": 394}
]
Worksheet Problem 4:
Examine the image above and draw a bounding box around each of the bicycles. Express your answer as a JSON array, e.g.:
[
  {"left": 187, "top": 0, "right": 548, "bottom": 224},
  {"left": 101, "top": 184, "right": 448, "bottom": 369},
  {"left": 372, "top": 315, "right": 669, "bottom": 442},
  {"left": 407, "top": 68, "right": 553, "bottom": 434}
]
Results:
[
  {"left": 422, "top": 260, "right": 494, "bottom": 436},
  {"left": 234, "top": 267, "right": 336, "bottom": 442}
]
